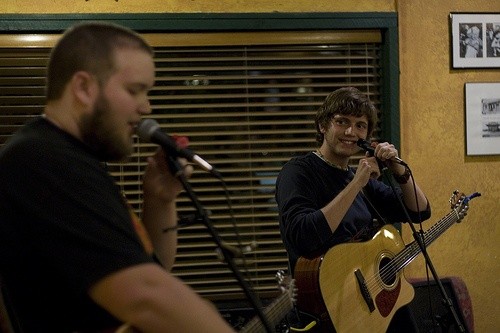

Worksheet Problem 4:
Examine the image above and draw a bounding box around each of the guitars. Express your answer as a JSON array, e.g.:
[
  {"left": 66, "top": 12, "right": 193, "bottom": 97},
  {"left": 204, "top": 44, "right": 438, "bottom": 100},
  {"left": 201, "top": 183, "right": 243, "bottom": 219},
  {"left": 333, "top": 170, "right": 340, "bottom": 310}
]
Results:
[
  {"left": 117, "top": 271, "right": 297, "bottom": 333},
  {"left": 293, "top": 190, "right": 482, "bottom": 333}
]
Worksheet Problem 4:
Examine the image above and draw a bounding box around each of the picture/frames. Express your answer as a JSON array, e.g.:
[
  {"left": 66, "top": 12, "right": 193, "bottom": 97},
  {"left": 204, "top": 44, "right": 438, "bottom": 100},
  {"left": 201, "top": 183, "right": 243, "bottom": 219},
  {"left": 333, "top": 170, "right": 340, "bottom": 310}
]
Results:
[{"left": 448, "top": 11, "right": 500, "bottom": 70}]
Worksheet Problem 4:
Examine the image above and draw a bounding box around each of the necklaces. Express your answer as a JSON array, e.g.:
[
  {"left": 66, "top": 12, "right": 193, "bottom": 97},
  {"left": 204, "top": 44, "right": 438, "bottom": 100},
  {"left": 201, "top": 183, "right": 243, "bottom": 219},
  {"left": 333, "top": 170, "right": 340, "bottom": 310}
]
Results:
[{"left": 316, "top": 149, "right": 349, "bottom": 170}]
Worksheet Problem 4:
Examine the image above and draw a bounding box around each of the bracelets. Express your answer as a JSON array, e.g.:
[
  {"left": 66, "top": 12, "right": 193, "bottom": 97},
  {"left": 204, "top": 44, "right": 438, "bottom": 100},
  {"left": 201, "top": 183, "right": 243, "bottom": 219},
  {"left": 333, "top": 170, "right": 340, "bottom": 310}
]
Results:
[{"left": 392, "top": 166, "right": 410, "bottom": 184}]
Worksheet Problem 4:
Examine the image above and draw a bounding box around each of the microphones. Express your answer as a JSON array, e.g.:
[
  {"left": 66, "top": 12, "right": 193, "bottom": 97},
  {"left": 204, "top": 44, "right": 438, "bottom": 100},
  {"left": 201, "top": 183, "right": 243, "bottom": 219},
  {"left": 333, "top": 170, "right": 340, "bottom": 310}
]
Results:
[
  {"left": 136, "top": 119, "right": 221, "bottom": 180},
  {"left": 356, "top": 137, "right": 408, "bottom": 166}
]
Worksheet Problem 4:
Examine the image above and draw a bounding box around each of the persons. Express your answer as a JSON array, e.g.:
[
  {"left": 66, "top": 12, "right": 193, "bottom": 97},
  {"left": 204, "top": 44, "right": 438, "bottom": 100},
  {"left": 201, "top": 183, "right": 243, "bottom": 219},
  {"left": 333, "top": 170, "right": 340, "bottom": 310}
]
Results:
[
  {"left": 276, "top": 87, "right": 430, "bottom": 333},
  {"left": 459, "top": 24, "right": 500, "bottom": 57},
  {"left": 0, "top": 22, "right": 235, "bottom": 333}
]
